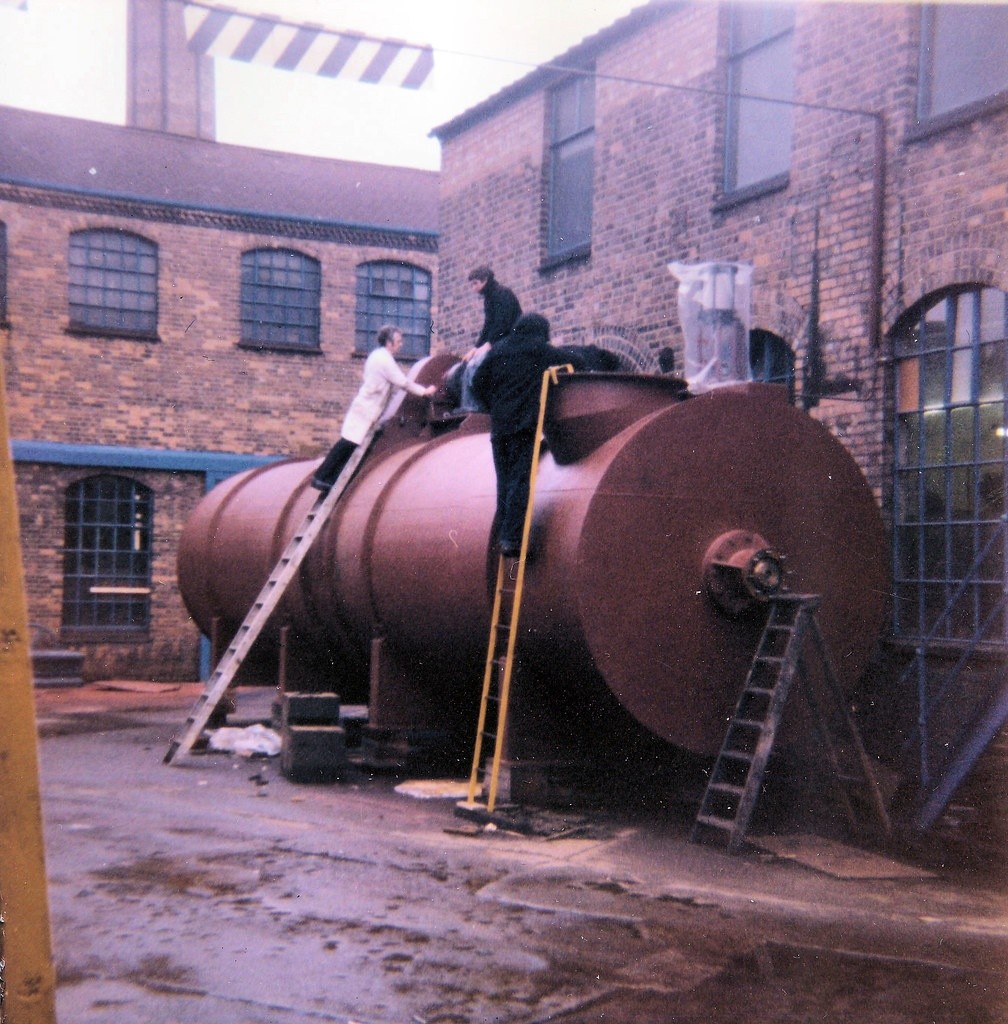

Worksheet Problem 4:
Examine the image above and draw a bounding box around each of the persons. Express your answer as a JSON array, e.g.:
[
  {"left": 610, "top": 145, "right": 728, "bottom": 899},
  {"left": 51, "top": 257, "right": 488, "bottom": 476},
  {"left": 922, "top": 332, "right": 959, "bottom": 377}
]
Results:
[
  {"left": 451, "top": 268, "right": 524, "bottom": 412},
  {"left": 310, "top": 325, "right": 436, "bottom": 488},
  {"left": 659, "top": 349, "right": 716, "bottom": 390},
  {"left": 472, "top": 312, "right": 590, "bottom": 555}
]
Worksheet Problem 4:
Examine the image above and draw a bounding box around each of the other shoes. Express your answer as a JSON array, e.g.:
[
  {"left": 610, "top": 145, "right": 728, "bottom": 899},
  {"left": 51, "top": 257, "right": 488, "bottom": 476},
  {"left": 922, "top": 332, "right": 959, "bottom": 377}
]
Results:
[
  {"left": 501, "top": 542, "right": 522, "bottom": 557},
  {"left": 310, "top": 477, "right": 333, "bottom": 492}
]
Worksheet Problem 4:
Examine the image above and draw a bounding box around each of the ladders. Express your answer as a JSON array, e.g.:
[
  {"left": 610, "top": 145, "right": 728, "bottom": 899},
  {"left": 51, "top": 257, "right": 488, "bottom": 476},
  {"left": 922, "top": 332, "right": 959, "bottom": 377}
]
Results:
[
  {"left": 687, "top": 587, "right": 894, "bottom": 857},
  {"left": 163, "top": 418, "right": 387, "bottom": 762},
  {"left": 467, "top": 367, "right": 609, "bottom": 807}
]
